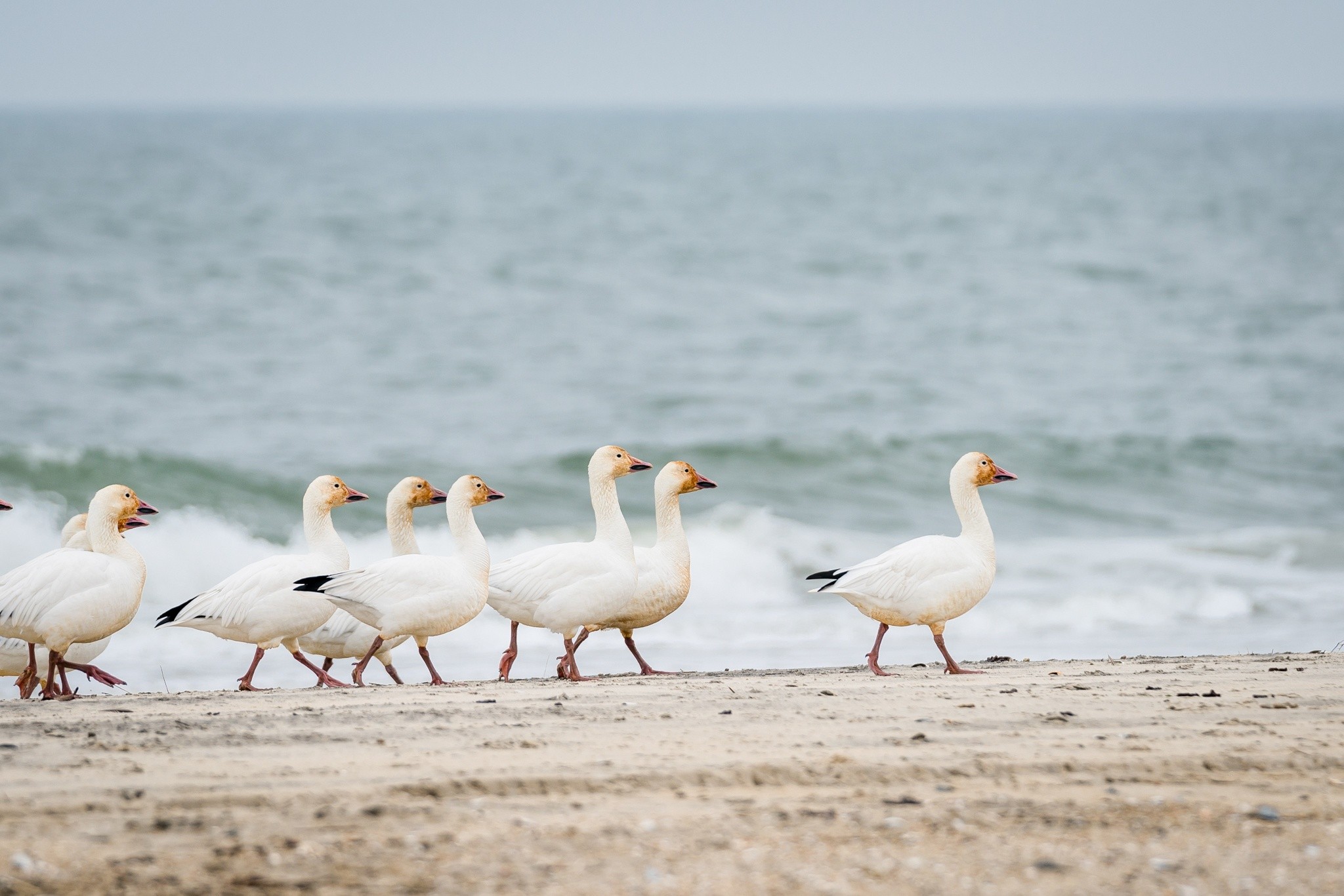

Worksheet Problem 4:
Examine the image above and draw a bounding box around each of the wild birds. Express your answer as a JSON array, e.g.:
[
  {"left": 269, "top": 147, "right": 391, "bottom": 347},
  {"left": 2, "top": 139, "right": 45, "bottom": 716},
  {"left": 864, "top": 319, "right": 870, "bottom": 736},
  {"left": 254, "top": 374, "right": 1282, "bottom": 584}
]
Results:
[
  {"left": 805, "top": 451, "right": 1019, "bottom": 674},
  {"left": 2, "top": 484, "right": 160, "bottom": 700},
  {"left": 154, "top": 441, "right": 716, "bottom": 686}
]
